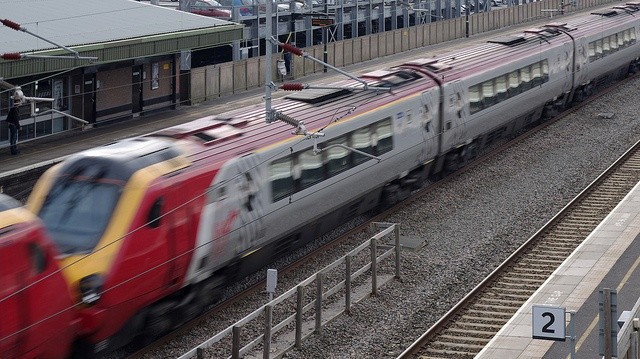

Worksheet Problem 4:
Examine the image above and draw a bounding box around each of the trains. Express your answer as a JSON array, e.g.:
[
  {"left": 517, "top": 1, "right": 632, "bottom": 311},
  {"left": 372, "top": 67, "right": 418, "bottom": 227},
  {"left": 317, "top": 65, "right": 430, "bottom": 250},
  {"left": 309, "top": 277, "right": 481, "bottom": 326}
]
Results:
[{"left": 0, "top": 1, "right": 640, "bottom": 359}]
